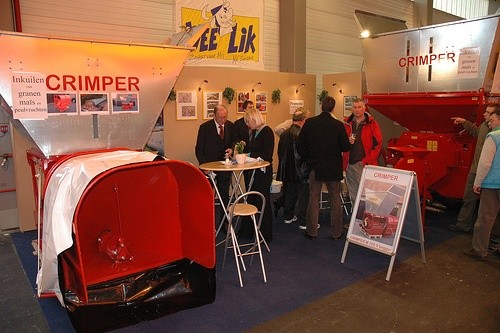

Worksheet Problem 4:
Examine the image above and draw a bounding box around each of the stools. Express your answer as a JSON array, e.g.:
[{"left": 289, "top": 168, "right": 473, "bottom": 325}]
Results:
[{"left": 220, "top": 190, "right": 267, "bottom": 289}]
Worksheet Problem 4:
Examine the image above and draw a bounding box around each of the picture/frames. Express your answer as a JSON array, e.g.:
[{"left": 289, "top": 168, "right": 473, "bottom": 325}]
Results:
[
  {"left": 342, "top": 94, "right": 358, "bottom": 119},
  {"left": 235, "top": 90, "right": 250, "bottom": 116},
  {"left": 288, "top": 99, "right": 304, "bottom": 115},
  {"left": 175, "top": 89, "right": 199, "bottom": 122},
  {"left": 202, "top": 89, "right": 223, "bottom": 121},
  {"left": 45, "top": 90, "right": 140, "bottom": 116},
  {"left": 253, "top": 91, "right": 268, "bottom": 115}
]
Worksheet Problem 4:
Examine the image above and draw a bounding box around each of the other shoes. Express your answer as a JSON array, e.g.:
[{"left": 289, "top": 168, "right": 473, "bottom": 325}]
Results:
[
  {"left": 218, "top": 223, "right": 231, "bottom": 235},
  {"left": 344, "top": 224, "right": 349, "bottom": 228},
  {"left": 448, "top": 225, "right": 471, "bottom": 232},
  {"left": 284, "top": 215, "right": 297, "bottom": 224},
  {"left": 273, "top": 200, "right": 281, "bottom": 218},
  {"left": 305, "top": 232, "right": 317, "bottom": 240},
  {"left": 299, "top": 224, "right": 320, "bottom": 229},
  {"left": 332, "top": 233, "right": 343, "bottom": 240},
  {"left": 464, "top": 247, "right": 487, "bottom": 257}
]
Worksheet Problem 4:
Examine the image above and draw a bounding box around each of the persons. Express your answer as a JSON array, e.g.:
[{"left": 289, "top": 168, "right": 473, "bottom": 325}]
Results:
[
  {"left": 465, "top": 109, "right": 500, "bottom": 262},
  {"left": 232, "top": 100, "right": 256, "bottom": 134},
  {"left": 193, "top": 104, "right": 238, "bottom": 236},
  {"left": 242, "top": 108, "right": 275, "bottom": 243},
  {"left": 447, "top": 102, "right": 500, "bottom": 232},
  {"left": 271, "top": 107, "right": 310, "bottom": 219},
  {"left": 275, "top": 110, "right": 321, "bottom": 230},
  {"left": 223, "top": 126, "right": 258, "bottom": 241},
  {"left": 342, "top": 98, "right": 383, "bottom": 230},
  {"left": 295, "top": 96, "right": 355, "bottom": 241}
]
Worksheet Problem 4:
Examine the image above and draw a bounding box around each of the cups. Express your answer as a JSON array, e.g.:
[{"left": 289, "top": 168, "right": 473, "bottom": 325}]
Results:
[
  {"left": 350, "top": 133, "right": 356, "bottom": 139},
  {"left": 235, "top": 153, "right": 245, "bottom": 164}
]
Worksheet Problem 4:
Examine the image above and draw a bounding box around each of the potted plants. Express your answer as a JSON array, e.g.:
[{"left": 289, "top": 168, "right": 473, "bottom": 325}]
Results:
[
  {"left": 223, "top": 86, "right": 234, "bottom": 105},
  {"left": 272, "top": 88, "right": 281, "bottom": 104},
  {"left": 168, "top": 87, "right": 176, "bottom": 102}
]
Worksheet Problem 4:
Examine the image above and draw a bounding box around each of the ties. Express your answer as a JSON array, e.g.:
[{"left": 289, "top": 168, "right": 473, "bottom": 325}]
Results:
[{"left": 218, "top": 125, "right": 224, "bottom": 140}]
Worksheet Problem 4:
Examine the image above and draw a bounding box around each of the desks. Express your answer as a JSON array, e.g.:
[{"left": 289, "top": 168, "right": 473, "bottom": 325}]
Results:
[{"left": 197, "top": 156, "right": 272, "bottom": 265}]
